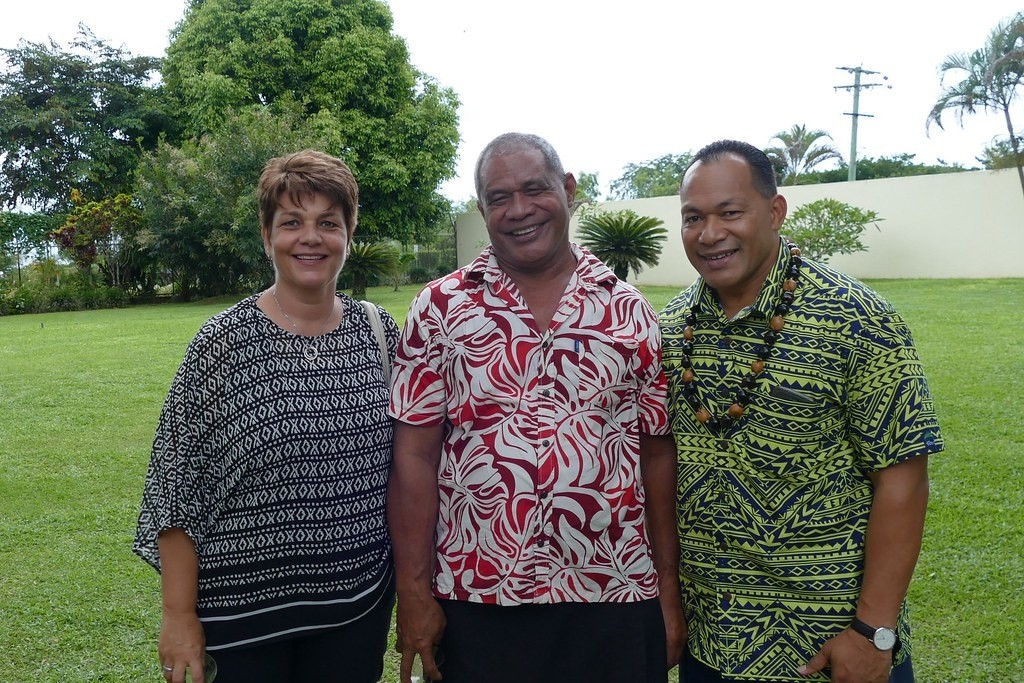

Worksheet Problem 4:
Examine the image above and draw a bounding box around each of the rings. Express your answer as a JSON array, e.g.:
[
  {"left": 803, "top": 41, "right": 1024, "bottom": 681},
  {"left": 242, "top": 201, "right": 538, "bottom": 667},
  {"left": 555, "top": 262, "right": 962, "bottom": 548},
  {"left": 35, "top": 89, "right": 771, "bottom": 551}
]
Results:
[{"left": 162, "top": 665, "right": 174, "bottom": 673}]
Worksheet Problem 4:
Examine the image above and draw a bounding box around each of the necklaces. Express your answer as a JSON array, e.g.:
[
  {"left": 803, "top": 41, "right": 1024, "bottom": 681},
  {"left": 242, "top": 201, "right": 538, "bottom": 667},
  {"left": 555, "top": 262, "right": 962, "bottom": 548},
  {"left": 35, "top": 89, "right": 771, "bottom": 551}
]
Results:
[
  {"left": 681, "top": 240, "right": 799, "bottom": 431},
  {"left": 270, "top": 284, "right": 335, "bottom": 360}
]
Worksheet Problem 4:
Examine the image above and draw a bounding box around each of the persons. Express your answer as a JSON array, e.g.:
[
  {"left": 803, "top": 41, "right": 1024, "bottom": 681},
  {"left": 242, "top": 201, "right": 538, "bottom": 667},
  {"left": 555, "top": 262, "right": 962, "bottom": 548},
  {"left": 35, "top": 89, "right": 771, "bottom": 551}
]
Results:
[
  {"left": 651, "top": 139, "right": 946, "bottom": 683},
  {"left": 131, "top": 146, "right": 402, "bottom": 683},
  {"left": 388, "top": 131, "right": 691, "bottom": 683}
]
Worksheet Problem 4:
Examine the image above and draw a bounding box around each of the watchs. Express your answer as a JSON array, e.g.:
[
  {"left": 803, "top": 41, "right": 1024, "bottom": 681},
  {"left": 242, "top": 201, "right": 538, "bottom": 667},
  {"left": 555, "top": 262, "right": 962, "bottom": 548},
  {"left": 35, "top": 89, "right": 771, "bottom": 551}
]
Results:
[{"left": 847, "top": 615, "right": 901, "bottom": 653}]
